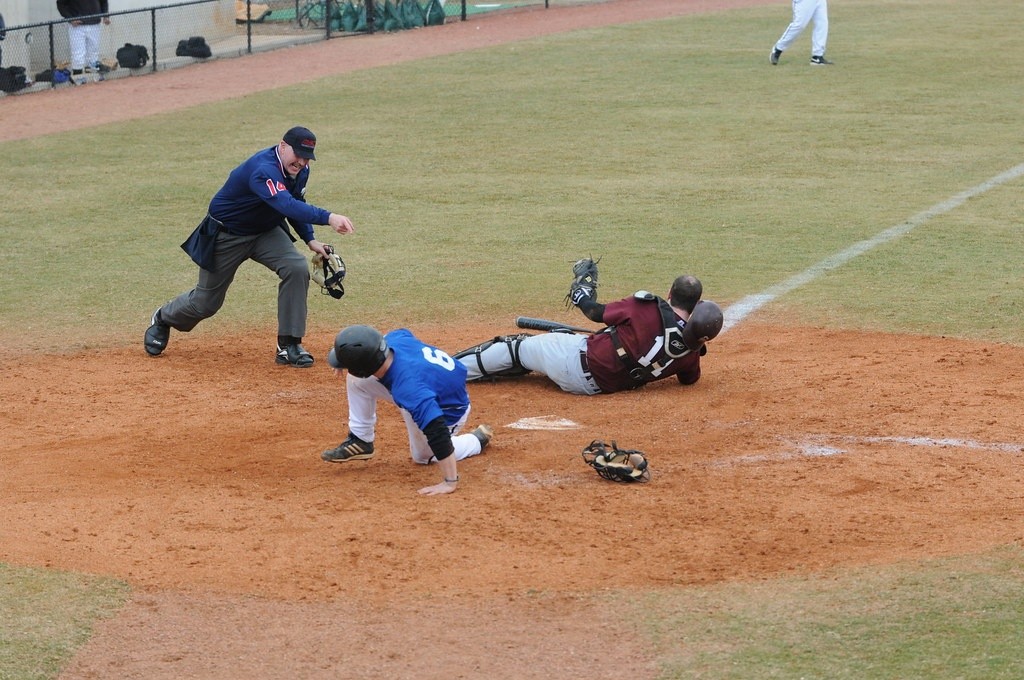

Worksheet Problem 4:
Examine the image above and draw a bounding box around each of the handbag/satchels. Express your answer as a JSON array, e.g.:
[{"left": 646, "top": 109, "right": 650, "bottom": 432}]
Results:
[
  {"left": 176, "top": 37, "right": 212, "bottom": 58},
  {"left": 35, "top": 69, "right": 74, "bottom": 87},
  {"left": 116, "top": 43, "right": 149, "bottom": 69},
  {"left": 86, "top": 61, "right": 110, "bottom": 72},
  {"left": 0, "top": 66, "right": 27, "bottom": 92}
]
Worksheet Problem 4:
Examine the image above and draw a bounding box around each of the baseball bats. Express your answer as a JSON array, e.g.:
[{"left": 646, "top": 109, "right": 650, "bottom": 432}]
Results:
[{"left": 515, "top": 316, "right": 597, "bottom": 334}]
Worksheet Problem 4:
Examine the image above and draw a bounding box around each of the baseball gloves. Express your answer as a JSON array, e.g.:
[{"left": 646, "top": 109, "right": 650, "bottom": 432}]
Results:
[{"left": 562, "top": 252, "right": 603, "bottom": 313}]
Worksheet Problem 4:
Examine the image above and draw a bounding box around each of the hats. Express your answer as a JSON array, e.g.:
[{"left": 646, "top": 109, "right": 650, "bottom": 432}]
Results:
[{"left": 283, "top": 126, "right": 317, "bottom": 161}]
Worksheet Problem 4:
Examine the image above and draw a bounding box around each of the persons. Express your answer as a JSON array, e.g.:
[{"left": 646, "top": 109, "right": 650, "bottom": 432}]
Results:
[
  {"left": 56, "top": 0, "right": 111, "bottom": 75},
  {"left": 452, "top": 258, "right": 723, "bottom": 396},
  {"left": 144, "top": 126, "right": 355, "bottom": 369},
  {"left": 321, "top": 325, "right": 493, "bottom": 496},
  {"left": 769, "top": 0, "right": 833, "bottom": 65}
]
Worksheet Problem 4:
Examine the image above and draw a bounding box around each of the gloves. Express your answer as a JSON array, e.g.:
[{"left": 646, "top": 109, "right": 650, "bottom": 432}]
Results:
[{"left": 572, "top": 287, "right": 592, "bottom": 305}]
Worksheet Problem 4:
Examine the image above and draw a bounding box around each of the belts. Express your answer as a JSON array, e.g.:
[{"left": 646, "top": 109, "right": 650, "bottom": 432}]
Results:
[
  {"left": 221, "top": 226, "right": 243, "bottom": 236},
  {"left": 579, "top": 350, "right": 601, "bottom": 392}
]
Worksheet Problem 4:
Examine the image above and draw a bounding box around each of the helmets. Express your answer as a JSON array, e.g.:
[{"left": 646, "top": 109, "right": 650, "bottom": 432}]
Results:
[
  {"left": 328, "top": 325, "right": 386, "bottom": 369},
  {"left": 683, "top": 301, "right": 723, "bottom": 352}
]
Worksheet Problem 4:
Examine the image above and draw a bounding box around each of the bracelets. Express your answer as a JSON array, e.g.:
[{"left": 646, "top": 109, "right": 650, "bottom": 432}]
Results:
[{"left": 445, "top": 476, "right": 459, "bottom": 482}]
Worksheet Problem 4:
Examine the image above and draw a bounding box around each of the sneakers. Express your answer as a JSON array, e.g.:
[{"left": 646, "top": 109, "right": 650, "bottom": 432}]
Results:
[
  {"left": 770, "top": 46, "right": 781, "bottom": 64},
  {"left": 472, "top": 424, "right": 493, "bottom": 448},
  {"left": 144, "top": 307, "right": 169, "bottom": 355},
  {"left": 810, "top": 56, "right": 833, "bottom": 65},
  {"left": 321, "top": 430, "right": 374, "bottom": 461},
  {"left": 275, "top": 343, "right": 313, "bottom": 367}
]
